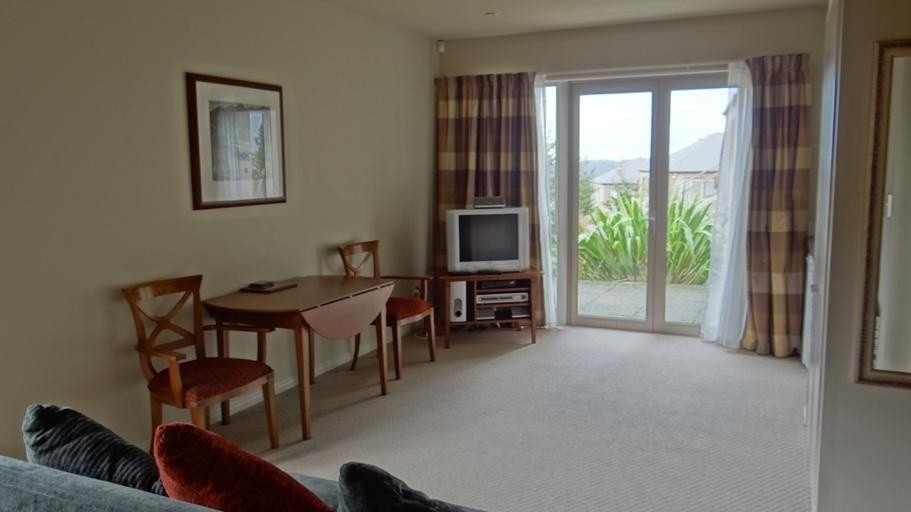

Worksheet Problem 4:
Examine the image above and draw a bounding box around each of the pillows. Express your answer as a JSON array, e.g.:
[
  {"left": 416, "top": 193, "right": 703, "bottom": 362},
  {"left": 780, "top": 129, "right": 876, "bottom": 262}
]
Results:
[
  {"left": 22, "top": 404, "right": 168, "bottom": 497},
  {"left": 153, "top": 421, "right": 333, "bottom": 512},
  {"left": 337, "top": 460, "right": 489, "bottom": 512}
]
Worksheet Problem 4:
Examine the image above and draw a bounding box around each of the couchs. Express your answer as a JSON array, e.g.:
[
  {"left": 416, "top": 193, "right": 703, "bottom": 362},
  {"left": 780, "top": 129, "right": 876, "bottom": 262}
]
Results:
[{"left": 0, "top": 451, "right": 337, "bottom": 512}]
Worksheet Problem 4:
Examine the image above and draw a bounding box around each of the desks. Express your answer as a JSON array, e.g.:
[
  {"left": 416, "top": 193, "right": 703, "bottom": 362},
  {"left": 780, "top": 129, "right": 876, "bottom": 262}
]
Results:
[{"left": 204, "top": 275, "right": 396, "bottom": 440}]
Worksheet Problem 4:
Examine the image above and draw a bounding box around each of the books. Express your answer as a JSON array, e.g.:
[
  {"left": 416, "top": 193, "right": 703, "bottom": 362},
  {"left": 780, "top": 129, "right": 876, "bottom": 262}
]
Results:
[
  {"left": 250, "top": 280, "right": 276, "bottom": 289},
  {"left": 477, "top": 307, "right": 496, "bottom": 319},
  {"left": 474, "top": 195, "right": 505, "bottom": 208},
  {"left": 241, "top": 279, "right": 298, "bottom": 293},
  {"left": 511, "top": 307, "right": 531, "bottom": 318}
]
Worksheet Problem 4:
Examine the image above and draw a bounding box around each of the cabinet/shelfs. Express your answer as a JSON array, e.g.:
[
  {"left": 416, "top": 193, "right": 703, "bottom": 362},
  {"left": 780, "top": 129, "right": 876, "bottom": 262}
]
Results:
[{"left": 439, "top": 265, "right": 544, "bottom": 348}]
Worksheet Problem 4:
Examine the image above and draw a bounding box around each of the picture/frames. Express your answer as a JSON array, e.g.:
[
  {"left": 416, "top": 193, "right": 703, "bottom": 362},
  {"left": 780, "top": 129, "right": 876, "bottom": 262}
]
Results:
[
  {"left": 856, "top": 38, "right": 911, "bottom": 389},
  {"left": 186, "top": 71, "right": 286, "bottom": 211}
]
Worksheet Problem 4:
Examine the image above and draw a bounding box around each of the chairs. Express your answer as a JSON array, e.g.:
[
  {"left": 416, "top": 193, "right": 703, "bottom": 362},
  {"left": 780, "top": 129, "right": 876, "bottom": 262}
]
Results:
[
  {"left": 120, "top": 274, "right": 279, "bottom": 450},
  {"left": 338, "top": 239, "right": 436, "bottom": 378}
]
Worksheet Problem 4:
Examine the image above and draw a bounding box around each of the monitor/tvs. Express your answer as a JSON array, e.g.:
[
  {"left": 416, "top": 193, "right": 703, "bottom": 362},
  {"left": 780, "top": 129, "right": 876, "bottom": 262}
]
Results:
[{"left": 445, "top": 207, "right": 531, "bottom": 274}]
